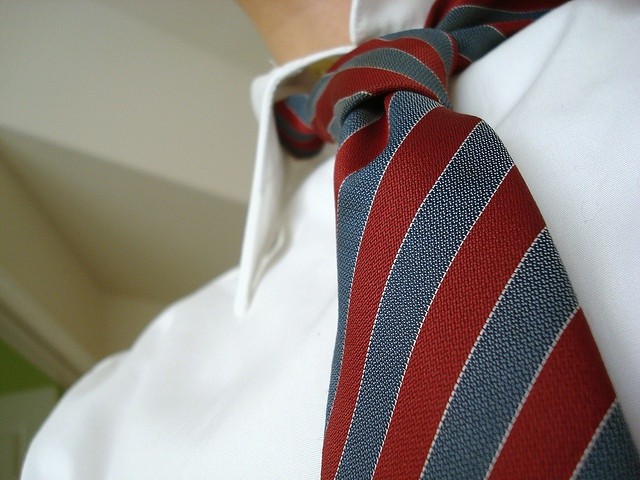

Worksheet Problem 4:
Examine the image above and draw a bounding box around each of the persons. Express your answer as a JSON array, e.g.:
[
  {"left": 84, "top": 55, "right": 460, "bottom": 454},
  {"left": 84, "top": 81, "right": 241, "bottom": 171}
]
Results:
[{"left": 18, "top": 1, "right": 640, "bottom": 480}]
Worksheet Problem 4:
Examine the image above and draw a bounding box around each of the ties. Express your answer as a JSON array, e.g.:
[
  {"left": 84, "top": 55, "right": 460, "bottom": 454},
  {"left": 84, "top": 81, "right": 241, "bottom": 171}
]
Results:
[{"left": 274, "top": 0, "right": 640, "bottom": 480}]
